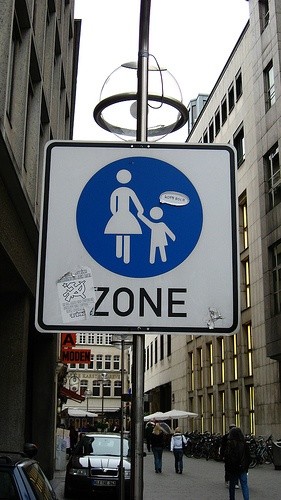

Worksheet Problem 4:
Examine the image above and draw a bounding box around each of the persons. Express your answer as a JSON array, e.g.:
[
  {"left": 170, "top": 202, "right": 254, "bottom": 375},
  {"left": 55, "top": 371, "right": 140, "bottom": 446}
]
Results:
[
  {"left": 170, "top": 427, "right": 189, "bottom": 474},
  {"left": 220, "top": 424, "right": 251, "bottom": 500},
  {"left": 149, "top": 425, "right": 165, "bottom": 473}
]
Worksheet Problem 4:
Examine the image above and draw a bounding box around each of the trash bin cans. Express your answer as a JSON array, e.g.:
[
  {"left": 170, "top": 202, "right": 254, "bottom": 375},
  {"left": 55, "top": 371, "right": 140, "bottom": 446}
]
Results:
[{"left": 272, "top": 441, "right": 281, "bottom": 470}]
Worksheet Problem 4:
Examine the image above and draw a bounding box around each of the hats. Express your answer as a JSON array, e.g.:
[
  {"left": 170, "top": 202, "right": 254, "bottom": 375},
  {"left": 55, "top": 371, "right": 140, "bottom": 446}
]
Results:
[
  {"left": 175, "top": 427, "right": 181, "bottom": 433},
  {"left": 147, "top": 423, "right": 153, "bottom": 427}
]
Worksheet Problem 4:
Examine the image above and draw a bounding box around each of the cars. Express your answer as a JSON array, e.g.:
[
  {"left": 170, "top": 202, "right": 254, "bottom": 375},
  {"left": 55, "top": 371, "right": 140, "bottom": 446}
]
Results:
[{"left": 65, "top": 432, "right": 147, "bottom": 500}]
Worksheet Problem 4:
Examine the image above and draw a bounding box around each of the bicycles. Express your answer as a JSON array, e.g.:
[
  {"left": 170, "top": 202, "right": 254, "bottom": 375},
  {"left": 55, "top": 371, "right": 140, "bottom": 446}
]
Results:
[{"left": 184, "top": 432, "right": 274, "bottom": 468}]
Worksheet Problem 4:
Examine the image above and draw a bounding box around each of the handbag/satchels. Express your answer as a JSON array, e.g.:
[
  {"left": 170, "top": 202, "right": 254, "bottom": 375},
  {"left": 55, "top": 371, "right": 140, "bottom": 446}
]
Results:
[{"left": 182, "top": 437, "right": 188, "bottom": 448}]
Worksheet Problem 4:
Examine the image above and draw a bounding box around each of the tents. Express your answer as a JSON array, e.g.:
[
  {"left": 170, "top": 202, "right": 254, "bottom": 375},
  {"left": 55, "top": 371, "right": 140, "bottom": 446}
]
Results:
[{"left": 144, "top": 409, "right": 198, "bottom": 431}]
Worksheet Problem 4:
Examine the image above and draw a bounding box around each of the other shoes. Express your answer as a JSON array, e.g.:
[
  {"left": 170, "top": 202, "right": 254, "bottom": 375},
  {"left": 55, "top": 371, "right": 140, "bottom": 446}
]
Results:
[
  {"left": 235, "top": 485, "right": 239, "bottom": 488},
  {"left": 225, "top": 481, "right": 228, "bottom": 488}
]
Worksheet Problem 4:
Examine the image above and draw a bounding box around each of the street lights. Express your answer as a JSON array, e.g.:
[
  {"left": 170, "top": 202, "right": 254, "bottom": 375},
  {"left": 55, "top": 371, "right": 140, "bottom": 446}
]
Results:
[{"left": 93, "top": 0, "right": 189, "bottom": 500}]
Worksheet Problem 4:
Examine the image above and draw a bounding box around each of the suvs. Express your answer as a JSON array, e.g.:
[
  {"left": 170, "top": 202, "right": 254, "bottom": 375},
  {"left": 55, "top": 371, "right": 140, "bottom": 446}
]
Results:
[{"left": 0, "top": 444, "right": 58, "bottom": 500}]
